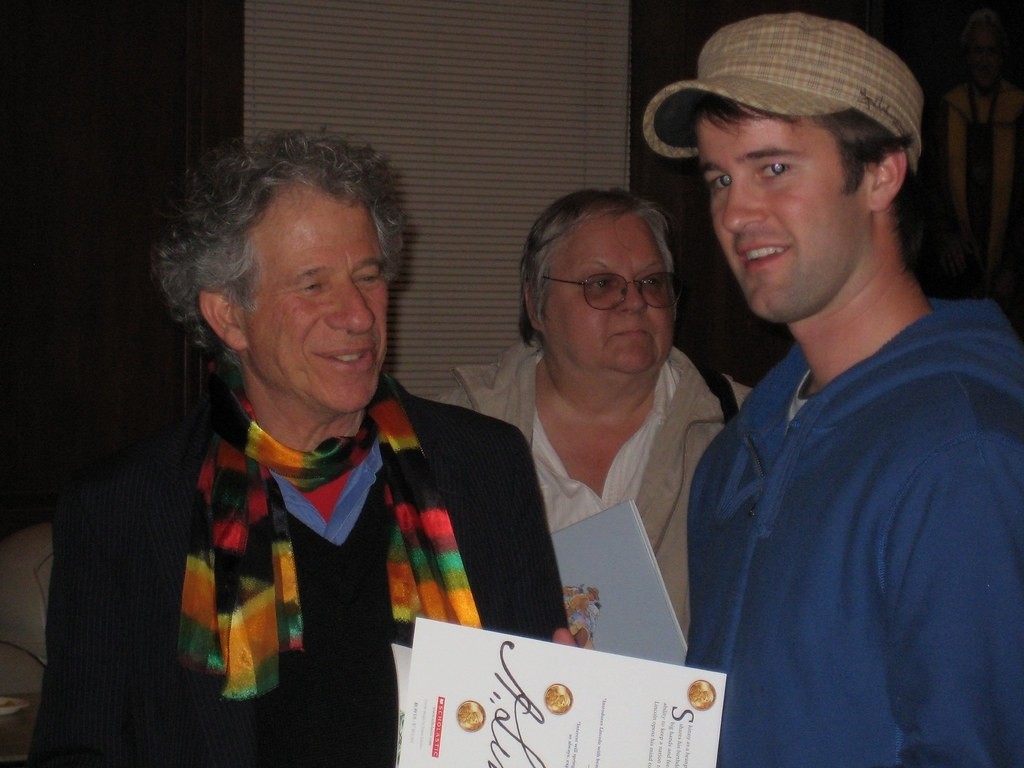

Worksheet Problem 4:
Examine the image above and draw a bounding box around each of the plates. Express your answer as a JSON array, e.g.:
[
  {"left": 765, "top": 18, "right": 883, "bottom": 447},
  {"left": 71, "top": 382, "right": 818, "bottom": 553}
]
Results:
[{"left": 0, "top": 697, "right": 30, "bottom": 715}]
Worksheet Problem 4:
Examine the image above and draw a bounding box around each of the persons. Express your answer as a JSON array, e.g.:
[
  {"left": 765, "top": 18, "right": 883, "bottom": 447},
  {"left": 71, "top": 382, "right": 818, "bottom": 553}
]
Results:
[
  {"left": 26, "top": 129, "right": 569, "bottom": 768},
  {"left": 419, "top": 188, "right": 755, "bottom": 644},
  {"left": 555, "top": 12, "right": 1024, "bottom": 768},
  {"left": 924, "top": 8, "right": 1024, "bottom": 339}
]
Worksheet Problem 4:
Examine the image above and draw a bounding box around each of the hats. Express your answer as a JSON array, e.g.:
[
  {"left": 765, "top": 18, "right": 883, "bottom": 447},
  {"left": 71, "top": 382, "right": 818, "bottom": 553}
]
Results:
[{"left": 643, "top": 13, "right": 923, "bottom": 173}]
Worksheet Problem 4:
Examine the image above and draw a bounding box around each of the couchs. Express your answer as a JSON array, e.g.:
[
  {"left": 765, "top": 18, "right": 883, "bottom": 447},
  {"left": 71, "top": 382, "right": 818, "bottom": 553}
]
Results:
[{"left": 0, "top": 520, "right": 54, "bottom": 768}]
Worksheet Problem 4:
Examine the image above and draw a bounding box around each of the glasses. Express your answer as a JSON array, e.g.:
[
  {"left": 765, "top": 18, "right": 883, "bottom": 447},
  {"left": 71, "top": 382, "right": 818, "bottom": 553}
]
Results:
[{"left": 541, "top": 273, "right": 682, "bottom": 311}]
